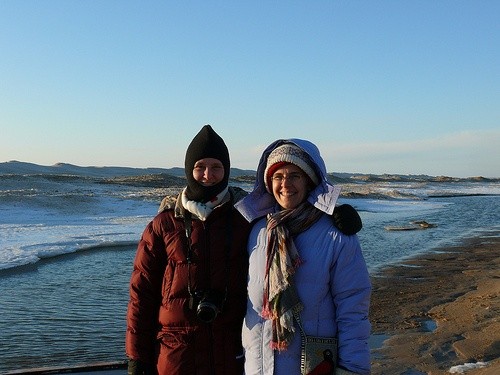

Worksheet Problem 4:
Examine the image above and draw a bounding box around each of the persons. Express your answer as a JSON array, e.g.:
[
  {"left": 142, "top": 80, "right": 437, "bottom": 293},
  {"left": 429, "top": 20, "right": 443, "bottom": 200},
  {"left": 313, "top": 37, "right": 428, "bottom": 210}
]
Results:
[
  {"left": 234, "top": 139, "right": 371, "bottom": 375},
  {"left": 126, "top": 125, "right": 362, "bottom": 375}
]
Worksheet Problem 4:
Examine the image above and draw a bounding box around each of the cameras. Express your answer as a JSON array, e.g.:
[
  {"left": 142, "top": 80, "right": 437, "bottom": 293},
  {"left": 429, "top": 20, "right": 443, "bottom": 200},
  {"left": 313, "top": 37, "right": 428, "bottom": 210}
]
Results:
[{"left": 187, "top": 288, "right": 228, "bottom": 322}]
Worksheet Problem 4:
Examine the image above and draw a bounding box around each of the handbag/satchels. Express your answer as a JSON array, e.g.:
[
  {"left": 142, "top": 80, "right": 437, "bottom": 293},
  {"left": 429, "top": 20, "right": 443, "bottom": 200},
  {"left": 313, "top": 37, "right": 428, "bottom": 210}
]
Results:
[{"left": 300, "top": 335, "right": 341, "bottom": 375}]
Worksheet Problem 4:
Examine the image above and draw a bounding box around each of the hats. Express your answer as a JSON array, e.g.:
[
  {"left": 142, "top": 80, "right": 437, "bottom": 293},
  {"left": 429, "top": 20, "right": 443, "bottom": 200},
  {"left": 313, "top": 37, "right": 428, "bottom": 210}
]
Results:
[
  {"left": 184, "top": 124, "right": 231, "bottom": 188},
  {"left": 259, "top": 145, "right": 323, "bottom": 197}
]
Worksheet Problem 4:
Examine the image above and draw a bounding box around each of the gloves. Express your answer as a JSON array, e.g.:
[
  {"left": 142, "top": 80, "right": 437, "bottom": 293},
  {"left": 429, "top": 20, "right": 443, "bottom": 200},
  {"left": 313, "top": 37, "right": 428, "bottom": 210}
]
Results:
[
  {"left": 331, "top": 200, "right": 365, "bottom": 236},
  {"left": 126, "top": 355, "right": 160, "bottom": 375}
]
